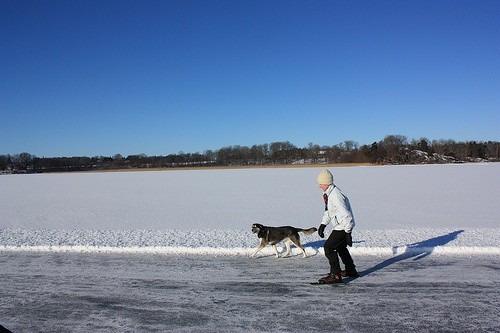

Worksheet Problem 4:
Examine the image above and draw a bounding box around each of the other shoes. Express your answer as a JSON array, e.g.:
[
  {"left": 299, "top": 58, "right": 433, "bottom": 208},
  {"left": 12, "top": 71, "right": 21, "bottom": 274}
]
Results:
[
  {"left": 341, "top": 268, "right": 358, "bottom": 276},
  {"left": 317, "top": 273, "right": 342, "bottom": 283}
]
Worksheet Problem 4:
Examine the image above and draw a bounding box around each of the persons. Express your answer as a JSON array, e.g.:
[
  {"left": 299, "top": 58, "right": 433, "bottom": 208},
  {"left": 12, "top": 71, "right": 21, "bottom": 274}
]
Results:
[{"left": 318, "top": 169, "right": 358, "bottom": 283}]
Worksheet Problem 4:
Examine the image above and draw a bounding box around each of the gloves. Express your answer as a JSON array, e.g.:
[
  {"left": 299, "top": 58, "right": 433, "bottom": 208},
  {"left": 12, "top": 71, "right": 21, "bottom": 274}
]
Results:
[
  {"left": 318, "top": 224, "right": 326, "bottom": 238},
  {"left": 345, "top": 232, "right": 352, "bottom": 247}
]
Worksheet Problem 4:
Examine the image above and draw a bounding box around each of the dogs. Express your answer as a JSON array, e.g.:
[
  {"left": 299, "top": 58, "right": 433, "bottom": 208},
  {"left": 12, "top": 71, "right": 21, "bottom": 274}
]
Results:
[{"left": 251, "top": 223, "right": 317, "bottom": 259}]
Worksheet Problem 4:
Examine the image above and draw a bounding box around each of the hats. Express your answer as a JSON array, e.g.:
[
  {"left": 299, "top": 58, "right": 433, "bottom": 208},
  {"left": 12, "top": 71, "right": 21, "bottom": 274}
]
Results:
[{"left": 317, "top": 170, "right": 333, "bottom": 184}]
